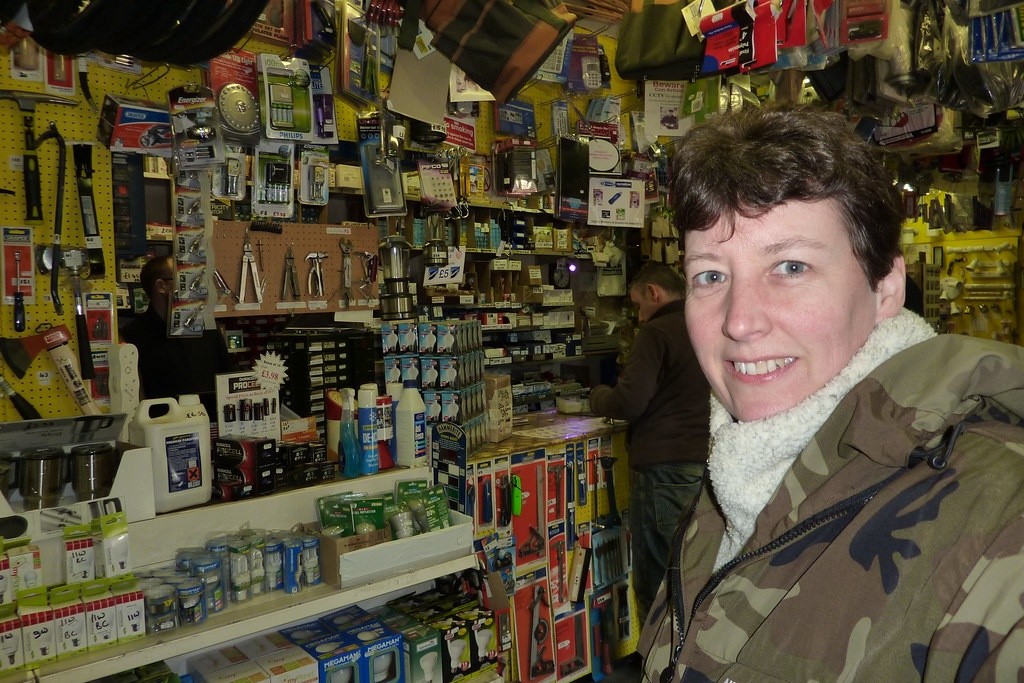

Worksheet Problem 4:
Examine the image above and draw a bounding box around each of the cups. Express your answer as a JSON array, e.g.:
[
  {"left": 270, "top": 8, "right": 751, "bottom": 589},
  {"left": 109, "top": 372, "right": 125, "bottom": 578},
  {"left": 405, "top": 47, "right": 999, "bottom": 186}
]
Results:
[
  {"left": 73, "top": 444, "right": 113, "bottom": 500},
  {"left": 18, "top": 448, "right": 67, "bottom": 511}
]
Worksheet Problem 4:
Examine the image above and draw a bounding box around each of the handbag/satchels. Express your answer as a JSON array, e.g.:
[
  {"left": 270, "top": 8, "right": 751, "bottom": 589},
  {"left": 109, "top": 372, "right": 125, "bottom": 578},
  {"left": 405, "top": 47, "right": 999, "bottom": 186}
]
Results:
[
  {"left": 615, "top": 0, "right": 707, "bottom": 81},
  {"left": 397, "top": 0, "right": 577, "bottom": 107}
]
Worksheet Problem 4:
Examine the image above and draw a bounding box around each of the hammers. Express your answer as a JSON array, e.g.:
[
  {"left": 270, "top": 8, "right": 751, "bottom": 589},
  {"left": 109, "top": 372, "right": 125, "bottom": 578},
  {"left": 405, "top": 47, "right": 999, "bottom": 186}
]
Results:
[{"left": 304, "top": 252, "right": 329, "bottom": 296}]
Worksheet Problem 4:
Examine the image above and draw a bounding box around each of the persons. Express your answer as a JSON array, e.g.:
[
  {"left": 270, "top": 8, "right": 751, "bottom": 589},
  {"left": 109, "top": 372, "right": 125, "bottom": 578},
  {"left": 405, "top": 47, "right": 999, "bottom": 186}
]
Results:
[
  {"left": 122, "top": 255, "right": 225, "bottom": 408},
  {"left": 633, "top": 100, "right": 1024, "bottom": 683},
  {"left": 586, "top": 260, "right": 712, "bottom": 629}
]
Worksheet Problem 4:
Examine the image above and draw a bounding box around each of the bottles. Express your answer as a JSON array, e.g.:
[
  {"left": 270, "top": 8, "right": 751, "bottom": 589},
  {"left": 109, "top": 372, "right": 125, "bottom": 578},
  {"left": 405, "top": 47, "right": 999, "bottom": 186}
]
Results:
[
  {"left": 133, "top": 394, "right": 212, "bottom": 513},
  {"left": 396, "top": 380, "right": 427, "bottom": 468}
]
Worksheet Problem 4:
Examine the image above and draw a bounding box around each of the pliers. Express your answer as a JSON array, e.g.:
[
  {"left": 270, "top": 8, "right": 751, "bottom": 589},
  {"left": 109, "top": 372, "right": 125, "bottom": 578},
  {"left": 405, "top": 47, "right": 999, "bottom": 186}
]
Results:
[
  {"left": 282, "top": 246, "right": 300, "bottom": 300},
  {"left": 308, "top": 259, "right": 320, "bottom": 298},
  {"left": 240, "top": 243, "right": 264, "bottom": 303}
]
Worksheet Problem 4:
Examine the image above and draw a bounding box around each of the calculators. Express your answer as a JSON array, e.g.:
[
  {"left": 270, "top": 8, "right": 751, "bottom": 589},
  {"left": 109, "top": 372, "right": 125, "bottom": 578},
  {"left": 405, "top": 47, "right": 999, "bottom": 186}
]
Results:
[
  {"left": 538, "top": 36, "right": 568, "bottom": 74},
  {"left": 581, "top": 56, "right": 602, "bottom": 89}
]
